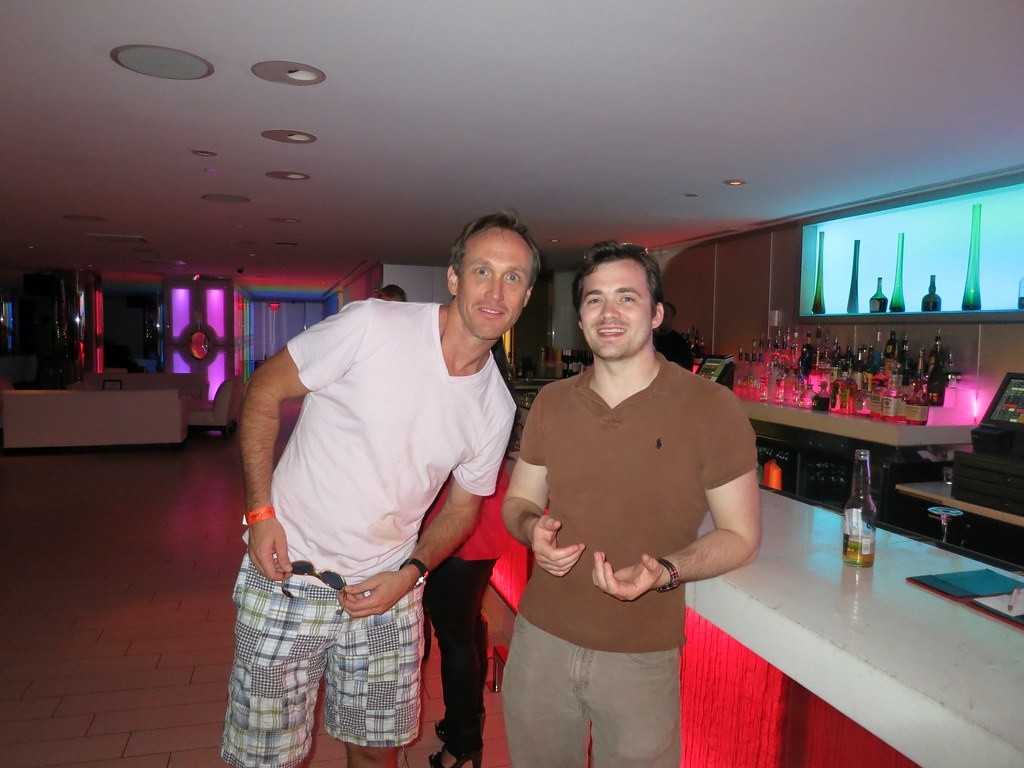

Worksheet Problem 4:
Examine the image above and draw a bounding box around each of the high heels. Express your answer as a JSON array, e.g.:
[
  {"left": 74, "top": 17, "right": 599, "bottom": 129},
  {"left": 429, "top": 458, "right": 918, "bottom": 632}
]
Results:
[
  {"left": 428, "top": 745, "right": 482, "bottom": 768},
  {"left": 435, "top": 722, "right": 448, "bottom": 743}
]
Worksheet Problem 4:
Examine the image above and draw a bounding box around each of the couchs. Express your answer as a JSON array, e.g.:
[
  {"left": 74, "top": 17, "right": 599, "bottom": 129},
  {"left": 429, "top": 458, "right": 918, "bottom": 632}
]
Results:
[
  {"left": 68, "top": 370, "right": 211, "bottom": 402},
  {"left": 3, "top": 375, "right": 191, "bottom": 453},
  {"left": 188, "top": 376, "right": 244, "bottom": 440}
]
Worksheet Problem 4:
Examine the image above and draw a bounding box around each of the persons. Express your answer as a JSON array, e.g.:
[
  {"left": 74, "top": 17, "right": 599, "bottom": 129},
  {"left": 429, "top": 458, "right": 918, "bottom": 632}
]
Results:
[
  {"left": 417, "top": 463, "right": 511, "bottom": 768},
  {"left": 219, "top": 211, "right": 540, "bottom": 768},
  {"left": 653, "top": 302, "right": 694, "bottom": 369},
  {"left": 378, "top": 284, "right": 406, "bottom": 302},
  {"left": 499, "top": 239, "right": 763, "bottom": 768}
]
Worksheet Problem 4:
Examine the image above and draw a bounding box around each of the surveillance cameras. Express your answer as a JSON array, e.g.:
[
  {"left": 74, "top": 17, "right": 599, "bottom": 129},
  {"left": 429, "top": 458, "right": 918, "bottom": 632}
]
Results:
[{"left": 237, "top": 269, "right": 244, "bottom": 273}]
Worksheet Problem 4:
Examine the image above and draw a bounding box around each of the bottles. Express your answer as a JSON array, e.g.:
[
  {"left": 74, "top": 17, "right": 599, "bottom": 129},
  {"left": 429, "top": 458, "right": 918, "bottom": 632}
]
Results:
[
  {"left": 842, "top": 449, "right": 876, "bottom": 568},
  {"left": 960, "top": 203, "right": 981, "bottom": 310},
  {"left": 521, "top": 392, "right": 530, "bottom": 409},
  {"left": 1018, "top": 278, "right": 1024, "bottom": 309},
  {"left": 888, "top": 232, "right": 905, "bottom": 312},
  {"left": 536, "top": 346, "right": 594, "bottom": 379},
  {"left": 847, "top": 240, "right": 860, "bottom": 313},
  {"left": 811, "top": 231, "right": 825, "bottom": 314},
  {"left": 869, "top": 277, "right": 888, "bottom": 313},
  {"left": 921, "top": 274, "right": 941, "bottom": 311},
  {"left": 681, "top": 325, "right": 704, "bottom": 357},
  {"left": 732, "top": 320, "right": 958, "bottom": 425},
  {"left": 518, "top": 360, "right": 525, "bottom": 381}
]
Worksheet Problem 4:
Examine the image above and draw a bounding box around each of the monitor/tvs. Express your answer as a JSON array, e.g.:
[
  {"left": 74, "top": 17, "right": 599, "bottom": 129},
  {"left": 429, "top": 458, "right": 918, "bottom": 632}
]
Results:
[
  {"left": 694, "top": 355, "right": 733, "bottom": 384},
  {"left": 979, "top": 372, "right": 1024, "bottom": 430}
]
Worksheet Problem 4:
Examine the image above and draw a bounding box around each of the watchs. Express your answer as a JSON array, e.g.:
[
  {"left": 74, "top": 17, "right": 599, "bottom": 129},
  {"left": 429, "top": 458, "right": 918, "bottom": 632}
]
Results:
[
  {"left": 399, "top": 558, "right": 430, "bottom": 589},
  {"left": 656, "top": 559, "right": 680, "bottom": 593}
]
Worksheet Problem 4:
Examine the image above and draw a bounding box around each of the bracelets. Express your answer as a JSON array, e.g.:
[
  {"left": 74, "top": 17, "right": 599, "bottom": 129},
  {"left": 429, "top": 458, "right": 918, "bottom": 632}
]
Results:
[{"left": 245, "top": 506, "right": 275, "bottom": 525}]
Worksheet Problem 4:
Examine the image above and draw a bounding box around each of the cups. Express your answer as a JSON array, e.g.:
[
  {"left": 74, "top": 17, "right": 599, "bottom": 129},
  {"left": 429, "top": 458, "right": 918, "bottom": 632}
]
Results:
[{"left": 524, "top": 370, "right": 533, "bottom": 382}]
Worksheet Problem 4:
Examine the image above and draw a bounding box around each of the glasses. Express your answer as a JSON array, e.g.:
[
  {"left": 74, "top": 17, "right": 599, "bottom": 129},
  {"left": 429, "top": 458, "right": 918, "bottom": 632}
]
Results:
[
  {"left": 281, "top": 562, "right": 348, "bottom": 616},
  {"left": 621, "top": 242, "right": 648, "bottom": 259}
]
